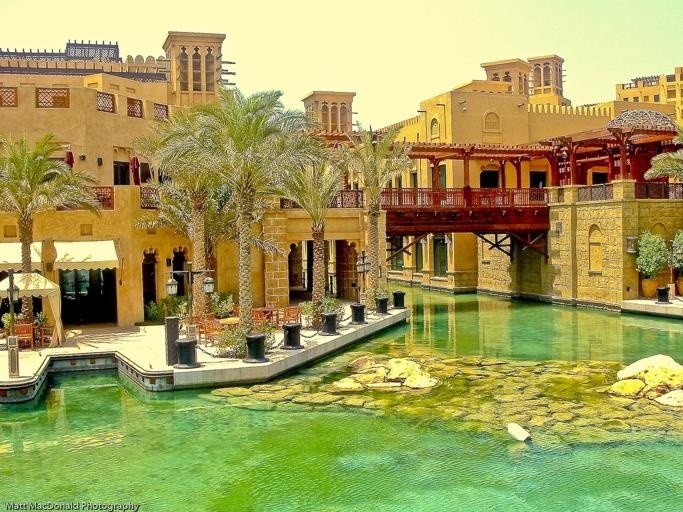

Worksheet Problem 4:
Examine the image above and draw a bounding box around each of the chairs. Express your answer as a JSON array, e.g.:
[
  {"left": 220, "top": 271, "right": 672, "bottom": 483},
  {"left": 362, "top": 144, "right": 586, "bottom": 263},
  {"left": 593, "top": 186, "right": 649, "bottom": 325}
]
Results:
[
  {"left": 186, "top": 301, "right": 302, "bottom": 347},
  {"left": 0, "top": 322, "right": 55, "bottom": 351}
]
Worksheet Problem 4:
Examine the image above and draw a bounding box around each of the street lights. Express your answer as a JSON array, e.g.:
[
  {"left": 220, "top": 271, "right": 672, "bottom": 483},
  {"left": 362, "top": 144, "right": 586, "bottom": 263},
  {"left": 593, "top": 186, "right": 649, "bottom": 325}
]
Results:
[
  {"left": 625, "top": 139, "right": 632, "bottom": 181},
  {"left": 5, "top": 267, "right": 19, "bottom": 336},
  {"left": 356, "top": 251, "right": 371, "bottom": 293},
  {"left": 561, "top": 146, "right": 569, "bottom": 186},
  {"left": 166, "top": 262, "right": 216, "bottom": 326}
]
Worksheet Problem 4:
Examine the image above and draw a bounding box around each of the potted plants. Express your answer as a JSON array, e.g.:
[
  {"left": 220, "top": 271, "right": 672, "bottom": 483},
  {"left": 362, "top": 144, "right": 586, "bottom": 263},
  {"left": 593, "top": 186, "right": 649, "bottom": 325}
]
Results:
[
  {"left": 667, "top": 228, "right": 683, "bottom": 295},
  {"left": 631, "top": 226, "right": 671, "bottom": 298}
]
